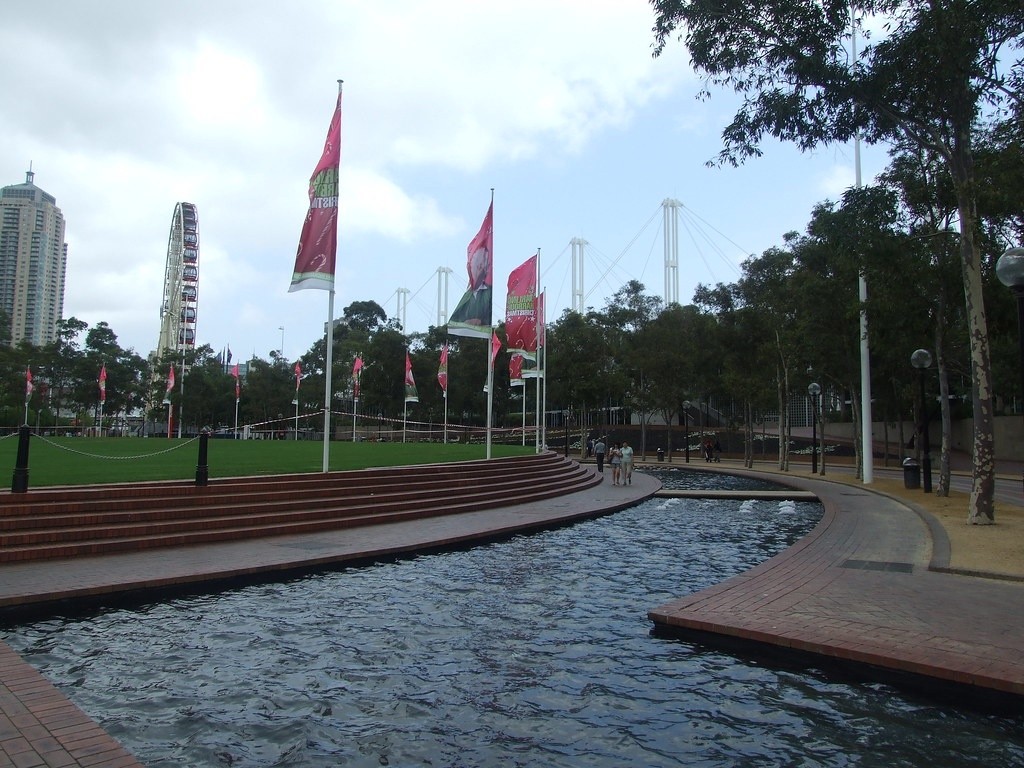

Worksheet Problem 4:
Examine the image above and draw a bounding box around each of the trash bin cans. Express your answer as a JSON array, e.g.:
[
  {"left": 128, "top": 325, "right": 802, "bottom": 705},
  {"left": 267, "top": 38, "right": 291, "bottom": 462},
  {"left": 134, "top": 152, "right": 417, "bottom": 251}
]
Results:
[
  {"left": 657, "top": 451, "right": 664, "bottom": 461},
  {"left": 903, "top": 457, "right": 922, "bottom": 489}
]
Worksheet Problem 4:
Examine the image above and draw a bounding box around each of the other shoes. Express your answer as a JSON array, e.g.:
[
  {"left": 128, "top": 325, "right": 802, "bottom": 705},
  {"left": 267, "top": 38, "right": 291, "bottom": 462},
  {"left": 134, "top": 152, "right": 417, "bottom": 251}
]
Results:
[
  {"left": 629, "top": 480, "right": 631, "bottom": 485},
  {"left": 623, "top": 482, "right": 626, "bottom": 485}
]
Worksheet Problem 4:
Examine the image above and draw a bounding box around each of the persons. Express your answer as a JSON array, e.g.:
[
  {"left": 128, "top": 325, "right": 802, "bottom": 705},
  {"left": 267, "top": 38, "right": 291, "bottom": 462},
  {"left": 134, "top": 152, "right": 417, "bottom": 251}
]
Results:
[
  {"left": 267, "top": 413, "right": 289, "bottom": 440},
  {"left": 370, "top": 432, "right": 386, "bottom": 443},
  {"left": 539, "top": 441, "right": 548, "bottom": 449},
  {"left": 713, "top": 440, "right": 721, "bottom": 463},
  {"left": 587, "top": 439, "right": 595, "bottom": 458},
  {"left": 609, "top": 441, "right": 623, "bottom": 485},
  {"left": 594, "top": 438, "right": 605, "bottom": 472},
  {"left": 41, "top": 430, "right": 82, "bottom": 437},
  {"left": 620, "top": 441, "right": 634, "bottom": 485},
  {"left": 703, "top": 438, "right": 713, "bottom": 463}
]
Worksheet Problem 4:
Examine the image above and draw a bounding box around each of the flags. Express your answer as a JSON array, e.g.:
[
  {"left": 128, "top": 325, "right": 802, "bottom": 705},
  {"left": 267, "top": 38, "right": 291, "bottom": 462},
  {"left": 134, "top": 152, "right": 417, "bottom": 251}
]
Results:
[
  {"left": 292, "top": 363, "right": 301, "bottom": 405},
  {"left": 24, "top": 368, "right": 32, "bottom": 406},
  {"left": 437, "top": 346, "right": 447, "bottom": 397},
  {"left": 521, "top": 293, "right": 544, "bottom": 378},
  {"left": 162, "top": 368, "right": 174, "bottom": 404},
  {"left": 447, "top": 200, "right": 492, "bottom": 339},
  {"left": 233, "top": 363, "right": 240, "bottom": 404},
  {"left": 287, "top": 92, "right": 342, "bottom": 293},
  {"left": 98, "top": 366, "right": 107, "bottom": 404},
  {"left": 405, "top": 353, "right": 419, "bottom": 402},
  {"left": 506, "top": 254, "right": 536, "bottom": 363},
  {"left": 352, "top": 356, "right": 364, "bottom": 397},
  {"left": 509, "top": 352, "right": 525, "bottom": 386},
  {"left": 483, "top": 332, "right": 501, "bottom": 392}
]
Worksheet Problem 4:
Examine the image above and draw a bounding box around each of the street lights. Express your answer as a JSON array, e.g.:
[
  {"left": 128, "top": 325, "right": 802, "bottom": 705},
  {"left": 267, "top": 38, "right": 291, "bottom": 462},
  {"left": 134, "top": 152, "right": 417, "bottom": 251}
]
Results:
[
  {"left": 682, "top": 400, "right": 691, "bottom": 463},
  {"left": 377, "top": 412, "right": 382, "bottom": 439},
  {"left": 630, "top": 367, "right": 645, "bottom": 422},
  {"left": 910, "top": 349, "right": 934, "bottom": 493},
  {"left": 563, "top": 409, "right": 570, "bottom": 458},
  {"left": 808, "top": 382, "right": 822, "bottom": 474}
]
